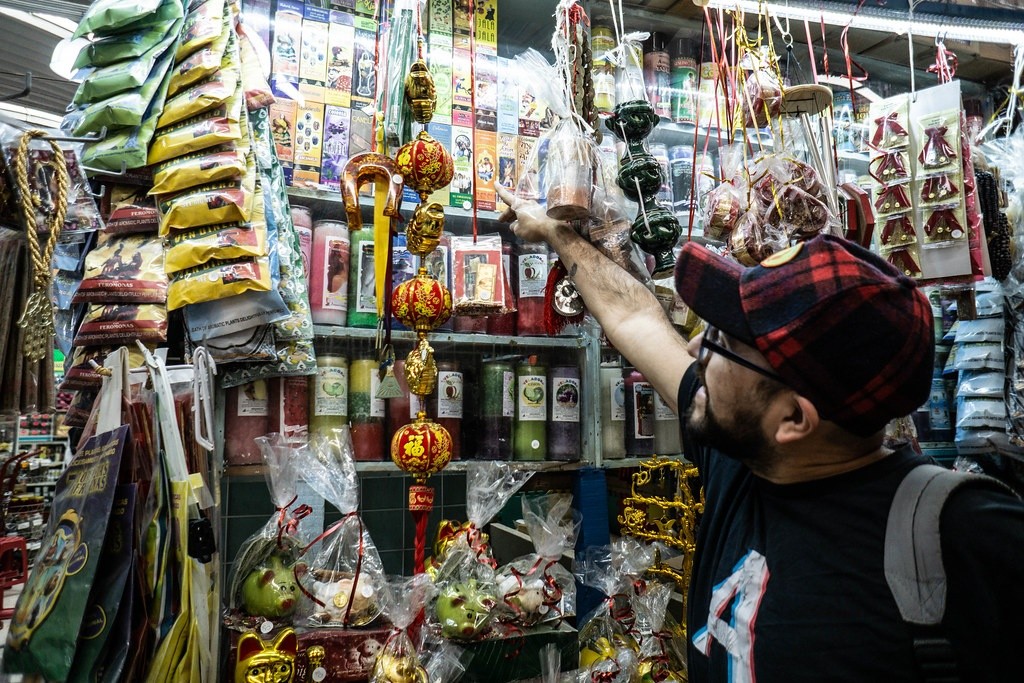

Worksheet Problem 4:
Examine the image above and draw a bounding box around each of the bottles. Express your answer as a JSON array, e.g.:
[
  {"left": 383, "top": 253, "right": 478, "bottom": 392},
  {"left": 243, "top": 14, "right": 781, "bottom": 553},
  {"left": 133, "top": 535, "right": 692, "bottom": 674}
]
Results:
[{"left": 226, "top": 203, "right": 683, "bottom": 465}]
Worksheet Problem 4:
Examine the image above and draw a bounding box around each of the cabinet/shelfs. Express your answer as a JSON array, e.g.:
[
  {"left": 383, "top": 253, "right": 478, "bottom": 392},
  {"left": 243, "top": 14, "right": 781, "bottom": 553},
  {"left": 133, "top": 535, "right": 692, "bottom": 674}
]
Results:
[{"left": 0, "top": 1, "right": 1024, "bottom": 683}]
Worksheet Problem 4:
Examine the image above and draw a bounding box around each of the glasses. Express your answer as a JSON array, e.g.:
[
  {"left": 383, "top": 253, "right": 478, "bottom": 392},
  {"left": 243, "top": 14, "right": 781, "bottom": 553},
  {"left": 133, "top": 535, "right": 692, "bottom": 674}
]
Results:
[{"left": 699, "top": 318, "right": 796, "bottom": 395}]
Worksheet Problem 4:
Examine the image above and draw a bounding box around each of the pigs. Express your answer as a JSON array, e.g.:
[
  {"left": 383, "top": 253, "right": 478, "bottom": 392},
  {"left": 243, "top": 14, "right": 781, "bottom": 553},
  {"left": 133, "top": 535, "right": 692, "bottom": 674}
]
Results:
[
  {"left": 374, "top": 654, "right": 423, "bottom": 683},
  {"left": 241, "top": 553, "right": 308, "bottom": 620},
  {"left": 435, "top": 579, "right": 496, "bottom": 642}
]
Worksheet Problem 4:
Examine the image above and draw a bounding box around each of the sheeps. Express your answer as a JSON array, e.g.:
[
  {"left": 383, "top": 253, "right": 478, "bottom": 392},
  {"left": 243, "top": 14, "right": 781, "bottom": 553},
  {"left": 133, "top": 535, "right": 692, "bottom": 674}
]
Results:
[{"left": 496, "top": 573, "right": 553, "bottom": 620}]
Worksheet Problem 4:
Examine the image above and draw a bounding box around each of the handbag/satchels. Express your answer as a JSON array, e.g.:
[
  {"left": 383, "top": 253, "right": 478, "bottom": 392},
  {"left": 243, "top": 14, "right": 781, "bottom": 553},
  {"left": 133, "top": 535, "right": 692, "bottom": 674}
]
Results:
[{"left": 2, "top": 348, "right": 216, "bottom": 683}]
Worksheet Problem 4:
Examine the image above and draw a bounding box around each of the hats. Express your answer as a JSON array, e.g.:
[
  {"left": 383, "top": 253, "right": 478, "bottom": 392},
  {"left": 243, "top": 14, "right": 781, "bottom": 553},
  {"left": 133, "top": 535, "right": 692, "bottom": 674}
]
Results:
[{"left": 674, "top": 238, "right": 935, "bottom": 440}]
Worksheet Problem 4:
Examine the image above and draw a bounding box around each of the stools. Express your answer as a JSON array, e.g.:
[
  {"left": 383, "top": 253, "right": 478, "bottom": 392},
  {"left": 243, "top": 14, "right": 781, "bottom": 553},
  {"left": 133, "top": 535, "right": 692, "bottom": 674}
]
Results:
[{"left": 0, "top": 536, "right": 28, "bottom": 630}]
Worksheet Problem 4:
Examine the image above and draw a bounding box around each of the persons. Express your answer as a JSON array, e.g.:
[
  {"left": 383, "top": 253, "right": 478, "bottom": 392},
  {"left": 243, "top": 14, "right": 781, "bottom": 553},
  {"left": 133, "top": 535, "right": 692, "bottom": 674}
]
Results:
[{"left": 494, "top": 181, "right": 1024, "bottom": 683}]
